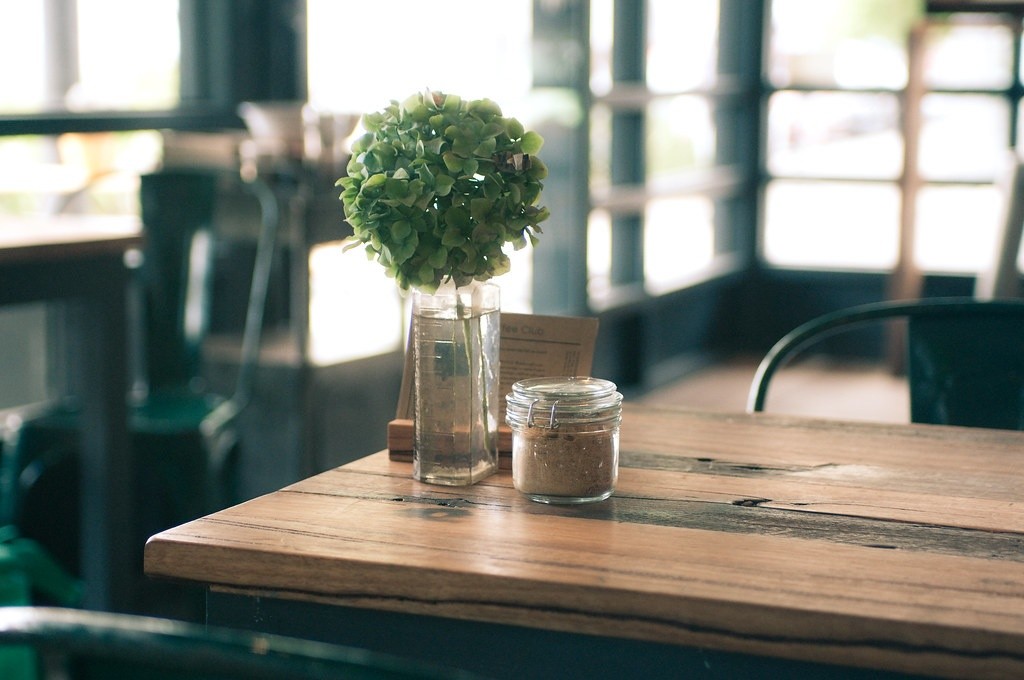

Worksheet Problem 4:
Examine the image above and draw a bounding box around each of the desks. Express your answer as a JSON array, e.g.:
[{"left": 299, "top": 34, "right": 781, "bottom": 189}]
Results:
[
  {"left": 142, "top": 402, "right": 1024, "bottom": 680},
  {"left": 0, "top": 213, "right": 146, "bottom": 680}
]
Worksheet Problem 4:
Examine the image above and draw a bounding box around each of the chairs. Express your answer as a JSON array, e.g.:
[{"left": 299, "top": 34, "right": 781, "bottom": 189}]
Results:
[
  {"left": 746, "top": 297, "right": 1024, "bottom": 431},
  {"left": 1, "top": 163, "right": 277, "bottom": 625}
]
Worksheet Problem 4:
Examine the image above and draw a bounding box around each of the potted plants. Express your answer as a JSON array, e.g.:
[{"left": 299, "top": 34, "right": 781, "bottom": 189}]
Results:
[{"left": 334, "top": 84, "right": 550, "bottom": 489}]
